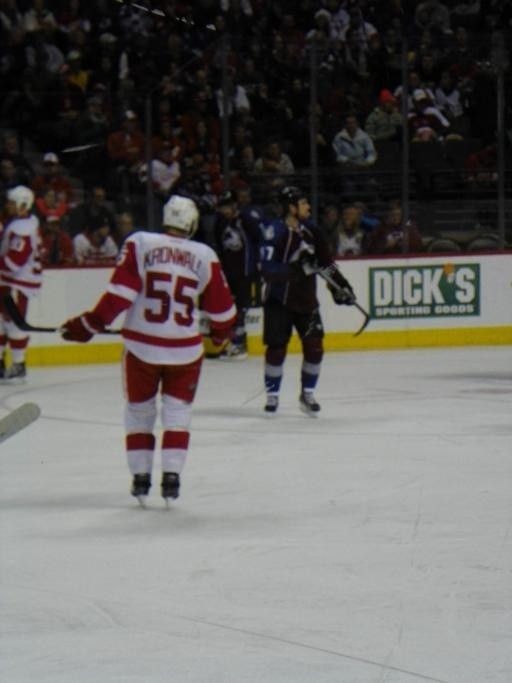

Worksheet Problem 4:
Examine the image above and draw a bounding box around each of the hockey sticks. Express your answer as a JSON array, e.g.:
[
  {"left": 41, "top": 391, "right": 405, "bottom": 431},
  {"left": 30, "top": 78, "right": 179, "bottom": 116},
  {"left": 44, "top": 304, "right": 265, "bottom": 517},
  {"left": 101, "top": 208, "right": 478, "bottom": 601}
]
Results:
[
  {"left": 2, "top": 290, "right": 125, "bottom": 334},
  {"left": 312, "top": 263, "right": 369, "bottom": 337}
]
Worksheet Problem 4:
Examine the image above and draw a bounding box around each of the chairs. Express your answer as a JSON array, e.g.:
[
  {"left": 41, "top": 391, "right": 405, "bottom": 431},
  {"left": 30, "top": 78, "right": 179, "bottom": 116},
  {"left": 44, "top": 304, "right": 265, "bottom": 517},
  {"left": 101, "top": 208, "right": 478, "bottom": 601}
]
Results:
[
  {"left": 464, "top": 233, "right": 507, "bottom": 252},
  {"left": 424, "top": 234, "right": 462, "bottom": 254}
]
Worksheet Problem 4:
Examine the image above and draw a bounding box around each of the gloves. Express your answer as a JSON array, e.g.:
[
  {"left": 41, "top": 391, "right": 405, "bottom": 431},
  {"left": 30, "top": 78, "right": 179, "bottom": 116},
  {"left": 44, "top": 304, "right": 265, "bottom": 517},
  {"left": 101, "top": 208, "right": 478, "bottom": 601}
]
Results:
[
  {"left": 61, "top": 312, "right": 103, "bottom": 342},
  {"left": 332, "top": 280, "right": 356, "bottom": 305}
]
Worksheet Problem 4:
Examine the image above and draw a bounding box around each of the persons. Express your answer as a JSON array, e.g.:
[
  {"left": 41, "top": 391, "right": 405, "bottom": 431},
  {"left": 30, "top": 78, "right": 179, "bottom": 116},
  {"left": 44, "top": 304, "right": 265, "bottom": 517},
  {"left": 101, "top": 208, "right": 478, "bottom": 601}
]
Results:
[
  {"left": 2, "top": 1, "right": 510, "bottom": 268},
  {"left": 210, "top": 193, "right": 261, "bottom": 361},
  {"left": 60, "top": 193, "right": 240, "bottom": 499},
  {"left": 255, "top": 190, "right": 358, "bottom": 418},
  {"left": 2, "top": 185, "right": 47, "bottom": 379}
]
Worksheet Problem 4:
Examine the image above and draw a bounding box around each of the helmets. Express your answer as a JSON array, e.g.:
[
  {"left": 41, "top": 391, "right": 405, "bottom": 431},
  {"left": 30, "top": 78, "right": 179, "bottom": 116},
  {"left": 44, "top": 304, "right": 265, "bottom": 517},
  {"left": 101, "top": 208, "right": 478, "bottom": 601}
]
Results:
[
  {"left": 8, "top": 186, "right": 34, "bottom": 210},
  {"left": 279, "top": 186, "right": 304, "bottom": 207},
  {"left": 162, "top": 195, "right": 199, "bottom": 239}
]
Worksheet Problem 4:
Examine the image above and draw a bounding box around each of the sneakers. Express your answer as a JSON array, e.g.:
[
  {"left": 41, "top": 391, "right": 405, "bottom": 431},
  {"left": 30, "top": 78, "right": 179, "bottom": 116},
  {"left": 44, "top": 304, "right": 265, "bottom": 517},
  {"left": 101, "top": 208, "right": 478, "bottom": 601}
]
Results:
[
  {"left": 300, "top": 392, "right": 319, "bottom": 411},
  {"left": 162, "top": 473, "right": 179, "bottom": 498},
  {"left": 132, "top": 473, "right": 150, "bottom": 495},
  {"left": 265, "top": 395, "right": 278, "bottom": 411}
]
